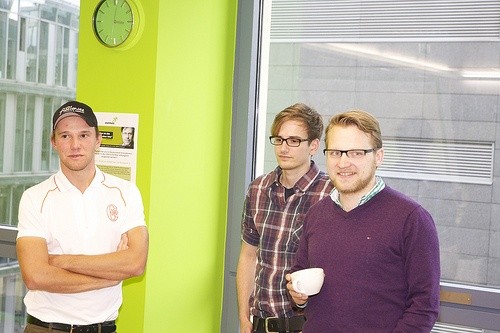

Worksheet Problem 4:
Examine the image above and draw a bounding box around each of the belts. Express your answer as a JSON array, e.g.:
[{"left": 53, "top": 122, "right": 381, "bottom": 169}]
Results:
[
  {"left": 25, "top": 312, "right": 117, "bottom": 333},
  {"left": 252, "top": 314, "right": 308, "bottom": 333}
]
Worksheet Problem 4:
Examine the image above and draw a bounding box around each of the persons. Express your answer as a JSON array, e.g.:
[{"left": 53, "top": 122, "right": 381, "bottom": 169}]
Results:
[
  {"left": 17, "top": 102, "right": 149, "bottom": 333},
  {"left": 121, "top": 127, "right": 134, "bottom": 148},
  {"left": 236, "top": 104, "right": 335, "bottom": 333},
  {"left": 285, "top": 111, "right": 439, "bottom": 333}
]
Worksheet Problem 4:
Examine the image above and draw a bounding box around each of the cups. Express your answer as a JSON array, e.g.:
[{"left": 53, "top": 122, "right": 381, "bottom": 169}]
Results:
[{"left": 290, "top": 268, "right": 324, "bottom": 296}]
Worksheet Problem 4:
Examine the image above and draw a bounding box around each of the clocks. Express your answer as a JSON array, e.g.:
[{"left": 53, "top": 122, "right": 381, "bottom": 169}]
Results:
[{"left": 92, "top": 0, "right": 140, "bottom": 49}]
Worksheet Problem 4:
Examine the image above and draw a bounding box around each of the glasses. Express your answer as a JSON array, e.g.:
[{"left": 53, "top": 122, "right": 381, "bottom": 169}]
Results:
[
  {"left": 269, "top": 136, "right": 313, "bottom": 147},
  {"left": 322, "top": 145, "right": 380, "bottom": 161}
]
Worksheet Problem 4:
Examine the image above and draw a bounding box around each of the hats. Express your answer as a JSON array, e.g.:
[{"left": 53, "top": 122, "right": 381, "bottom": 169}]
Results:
[{"left": 52, "top": 100, "right": 97, "bottom": 132}]
